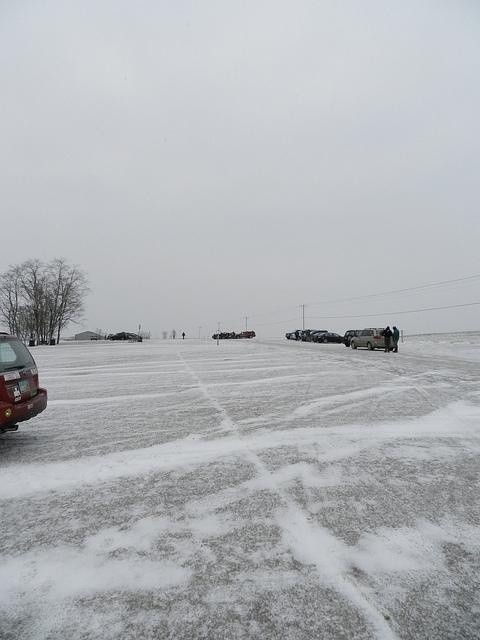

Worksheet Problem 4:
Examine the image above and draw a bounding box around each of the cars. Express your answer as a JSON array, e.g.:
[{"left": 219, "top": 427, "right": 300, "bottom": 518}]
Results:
[
  {"left": 213, "top": 331, "right": 256, "bottom": 339},
  {"left": 285, "top": 327, "right": 394, "bottom": 351},
  {"left": 0, "top": 331, "right": 48, "bottom": 434}
]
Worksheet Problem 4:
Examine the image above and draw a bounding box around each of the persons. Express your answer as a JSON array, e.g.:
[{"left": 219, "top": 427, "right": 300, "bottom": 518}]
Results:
[
  {"left": 381, "top": 325, "right": 393, "bottom": 352},
  {"left": 182, "top": 332, "right": 186, "bottom": 339},
  {"left": 391, "top": 325, "right": 400, "bottom": 353}
]
